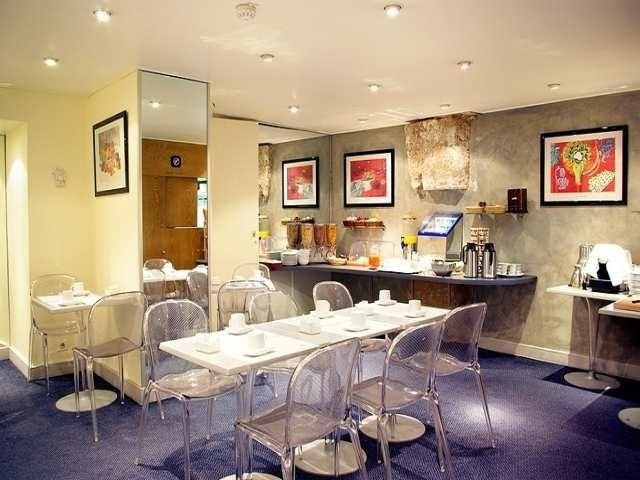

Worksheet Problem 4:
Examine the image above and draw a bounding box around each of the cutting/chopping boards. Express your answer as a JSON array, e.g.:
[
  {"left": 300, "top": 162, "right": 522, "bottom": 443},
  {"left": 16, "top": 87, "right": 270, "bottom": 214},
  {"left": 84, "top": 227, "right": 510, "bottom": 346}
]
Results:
[{"left": 613, "top": 297, "right": 640, "bottom": 312}]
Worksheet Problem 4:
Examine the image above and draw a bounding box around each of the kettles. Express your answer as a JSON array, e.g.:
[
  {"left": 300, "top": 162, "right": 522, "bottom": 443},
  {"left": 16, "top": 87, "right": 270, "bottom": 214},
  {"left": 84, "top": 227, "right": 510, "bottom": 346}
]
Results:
[
  {"left": 482, "top": 242, "right": 497, "bottom": 279},
  {"left": 462, "top": 242, "right": 478, "bottom": 278}
]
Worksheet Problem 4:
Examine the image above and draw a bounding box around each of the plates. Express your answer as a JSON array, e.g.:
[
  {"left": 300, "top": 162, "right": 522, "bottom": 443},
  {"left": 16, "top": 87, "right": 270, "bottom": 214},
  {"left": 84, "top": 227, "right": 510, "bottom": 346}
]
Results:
[
  {"left": 495, "top": 273, "right": 524, "bottom": 277},
  {"left": 310, "top": 310, "right": 335, "bottom": 318},
  {"left": 375, "top": 300, "right": 397, "bottom": 306},
  {"left": 485, "top": 204, "right": 506, "bottom": 215},
  {"left": 226, "top": 327, "right": 253, "bottom": 336},
  {"left": 242, "top": 349, "right": 273, "bottom": 356},
  {"left": 466, "top": 207, "right": 484, "bottom": 214},
  {"left": 57, "top": 300, "right": 81, "bottom": 306},
  {"left": 330, "top": 259, "right": 345, "bottom": 265},
  {"left": 403, "top": 311, "right": 425, "bottom": 318},
  {"left": 266, "top": 251, "right": 281, "bottom": 259},
  {"left": 345, "top": 326, "right": 370, "bottom": 331},
  {"left": 73, "top": 290, "right": 90, "bottom": 297}
]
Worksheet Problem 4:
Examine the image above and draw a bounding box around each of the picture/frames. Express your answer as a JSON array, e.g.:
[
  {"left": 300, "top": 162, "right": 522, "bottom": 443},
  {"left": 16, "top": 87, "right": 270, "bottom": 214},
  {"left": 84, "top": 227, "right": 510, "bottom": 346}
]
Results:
[
  {"left": 540, "top": 125, "right": 628, "bottom": 206},
  {"left": 282, "top": 156, "right": 319, "bottom": 208},
  {"left": 93, "top": 111, "right": 129, "bottom": 196},
  {"left": 344, "top": 149, "right": 394, "bottom": 207}
]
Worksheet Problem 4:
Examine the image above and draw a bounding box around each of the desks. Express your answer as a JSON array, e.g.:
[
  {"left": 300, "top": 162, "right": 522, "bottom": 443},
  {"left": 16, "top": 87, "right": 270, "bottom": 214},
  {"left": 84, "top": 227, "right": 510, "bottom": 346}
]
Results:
[
  {"left": 598, "top": 293, "right": 640, "bottom": 429},
  {"left": 33, "top": 291, "right": 117, "bottom": 413},
  {"left": 546, "top": 285, "right": 629, "bottom": 390}
]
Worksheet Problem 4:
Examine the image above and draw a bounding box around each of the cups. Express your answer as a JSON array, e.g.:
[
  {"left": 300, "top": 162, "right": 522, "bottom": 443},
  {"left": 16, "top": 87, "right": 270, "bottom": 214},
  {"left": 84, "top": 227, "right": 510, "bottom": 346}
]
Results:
[
  {"left": 71, "top": 282, "right": 84, "bottom": 294},
  {"left": 315, "top": 299, "right": 330, "bottom": 314},
  {"left": 350, "top": 311, "right": 363, "bottom": 327},
  {"left": 247, "top": 330, "right": 268, "bottom": 352},
  {"left": 62, "top": 290, "right": 73, "bottom": 302},
  {"left": 229, "top": 312, "right": 247, "bottom": 330},
  {"left": 368, "top": 246, "right": 381, "bottom": 267},
  {"left": 299, "top": 251, "right": 310, "bottom": 265},
  {"left": 379, "top": 289, "right": 392, "bottom": 304},
  {"left": 497, "top": 263, "right": 525, "bottom": 274},
  {"left": 408, "top": 299, "right": 422, "bottom": 315}
]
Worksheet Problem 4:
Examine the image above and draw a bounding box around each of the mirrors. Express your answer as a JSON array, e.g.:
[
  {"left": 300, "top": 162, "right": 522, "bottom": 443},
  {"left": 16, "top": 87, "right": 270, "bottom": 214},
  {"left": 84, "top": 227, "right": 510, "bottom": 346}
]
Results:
[
  {"left": 258, "top": 123, "right": 330, "bottom": 258},
  {"left": 139, "top": 69, "right": 208, "bottom": 385}
]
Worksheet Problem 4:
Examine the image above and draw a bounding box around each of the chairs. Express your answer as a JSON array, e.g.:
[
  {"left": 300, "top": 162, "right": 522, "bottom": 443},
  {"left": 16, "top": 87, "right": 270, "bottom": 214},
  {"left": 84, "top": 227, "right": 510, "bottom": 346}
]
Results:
[
  {"left": 72, "top": 290, "right": 147, "bottom": 443},
  {"left": 144, "top": 257, "right": 208, "bottom": 309},
  {"left": 30, "top": 275, "right": 86, "bottom": 397}
]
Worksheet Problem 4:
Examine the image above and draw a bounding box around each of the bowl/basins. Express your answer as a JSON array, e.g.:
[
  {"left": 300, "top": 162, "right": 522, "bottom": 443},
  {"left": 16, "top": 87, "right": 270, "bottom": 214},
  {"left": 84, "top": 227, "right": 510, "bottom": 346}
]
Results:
[{"left": 431, "top": 260, "right": 456, "bottom": 275}]
